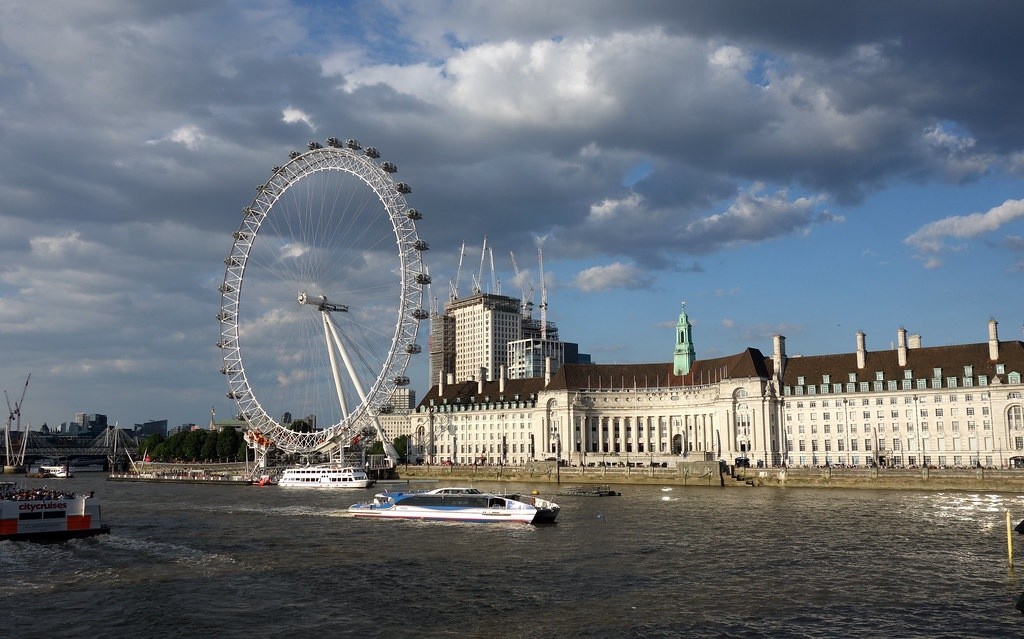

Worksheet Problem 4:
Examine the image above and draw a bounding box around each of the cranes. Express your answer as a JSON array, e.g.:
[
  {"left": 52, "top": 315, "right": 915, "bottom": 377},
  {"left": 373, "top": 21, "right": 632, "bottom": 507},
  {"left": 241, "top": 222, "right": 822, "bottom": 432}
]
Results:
[
  {"left": 425, "top": 235, "right": 548, "bottom": 340},
  {"left": 3, "top": 372, "right": 31, "bottom": 431}
]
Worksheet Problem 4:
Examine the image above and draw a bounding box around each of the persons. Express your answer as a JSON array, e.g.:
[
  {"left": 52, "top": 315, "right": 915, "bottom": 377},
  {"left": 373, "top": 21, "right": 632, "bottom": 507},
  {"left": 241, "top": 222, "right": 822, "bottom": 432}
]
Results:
[
  {"left": 0, "top": 486, "right": 77, "bottom": 500},
  {"left": 143, "top": 453, "right": 151, "bottom": 463}
]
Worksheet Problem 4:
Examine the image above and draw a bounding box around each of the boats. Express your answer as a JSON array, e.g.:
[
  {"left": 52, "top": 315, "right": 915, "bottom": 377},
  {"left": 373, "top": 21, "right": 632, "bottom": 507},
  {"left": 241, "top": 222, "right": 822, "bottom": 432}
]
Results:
[
  {"left": 276, "top": 465, "right": 377, "bottom": 490},
  {"left": 41, "top": 464, "right": 73, "bottom": 479},
  {"left": 347, "top": 487, "right": 561, "bottom": 525},
  {"left": 570, "top": 484, "right": 622, "bottom": 500},
  {"left": 0, "top": 485, "right": 108, "bottom": 542}
]
